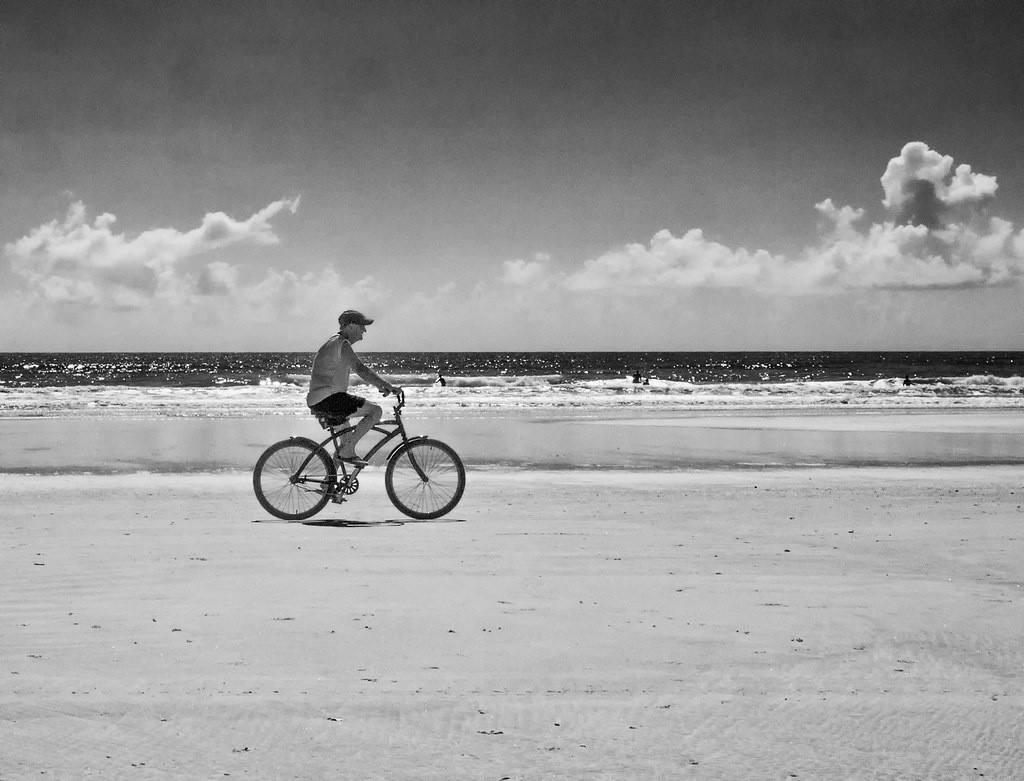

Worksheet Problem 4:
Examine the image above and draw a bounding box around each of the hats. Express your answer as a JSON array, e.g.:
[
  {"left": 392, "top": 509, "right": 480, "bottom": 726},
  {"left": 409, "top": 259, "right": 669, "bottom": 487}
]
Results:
[{"left": 338, "top": 310, "right": 374, "bottom": 325}]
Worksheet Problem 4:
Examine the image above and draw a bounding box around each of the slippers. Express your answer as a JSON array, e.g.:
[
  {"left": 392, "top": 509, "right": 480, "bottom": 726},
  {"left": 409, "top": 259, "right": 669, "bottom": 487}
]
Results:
[
  {"left": 315, "top": 486, "right": 348, "bottom": 501},
  {"left": 334, "top": 452, "right": 369, "bottom": 466}
]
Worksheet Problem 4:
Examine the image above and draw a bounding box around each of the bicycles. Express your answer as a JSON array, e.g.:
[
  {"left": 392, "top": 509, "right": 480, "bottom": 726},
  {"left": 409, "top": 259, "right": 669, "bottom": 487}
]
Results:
[{"left": 252, "top": 385, "right": 465, "bottom": 522}]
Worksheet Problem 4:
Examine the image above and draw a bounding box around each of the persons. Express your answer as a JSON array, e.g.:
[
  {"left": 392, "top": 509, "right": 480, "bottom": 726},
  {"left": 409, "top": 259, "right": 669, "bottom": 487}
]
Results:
[
  {"left": 633, "top": 370, "right": 649, "bottom": 385},
  {"left": 435, "top": 374, "right": 446, "bottom": 387},
  {"left": 306, "top": 309, "right": 402, "bottom": 502},
  {"left": 903, "top": 375, "right": 915, "bottom": 386}
]
